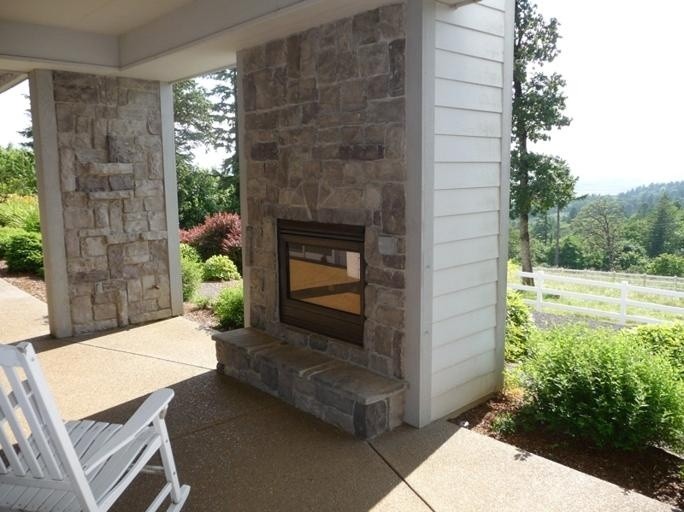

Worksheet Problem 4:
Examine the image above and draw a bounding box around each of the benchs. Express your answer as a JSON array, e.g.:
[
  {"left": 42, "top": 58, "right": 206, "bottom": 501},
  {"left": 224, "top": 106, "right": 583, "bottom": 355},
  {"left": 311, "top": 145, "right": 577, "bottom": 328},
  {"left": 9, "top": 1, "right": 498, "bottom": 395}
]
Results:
[{"left": 210, "top": 328, "right": 407, "bottom": 443}]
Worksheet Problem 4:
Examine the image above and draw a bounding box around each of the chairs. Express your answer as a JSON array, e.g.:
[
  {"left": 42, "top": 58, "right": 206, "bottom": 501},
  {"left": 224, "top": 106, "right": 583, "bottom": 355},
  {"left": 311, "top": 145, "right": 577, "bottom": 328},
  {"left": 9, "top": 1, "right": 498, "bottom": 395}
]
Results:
[{"left": 0, "top": 342, "right": 191, "bottom": 512}]
